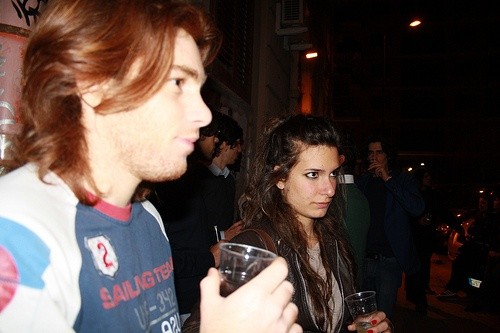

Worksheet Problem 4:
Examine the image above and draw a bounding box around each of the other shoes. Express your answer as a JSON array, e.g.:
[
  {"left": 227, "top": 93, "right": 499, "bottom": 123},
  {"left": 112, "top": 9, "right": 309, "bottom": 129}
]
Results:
[
  {"left": 426, "top": 288, "right": 437, "bottom": 294},
  {"left": 438, "top": 290, "right": 450, "bottom": 297}
]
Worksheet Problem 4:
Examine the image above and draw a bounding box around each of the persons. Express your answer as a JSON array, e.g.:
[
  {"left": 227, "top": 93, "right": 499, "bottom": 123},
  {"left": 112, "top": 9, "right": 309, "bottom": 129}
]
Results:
[
  {"left": 147, "top": 107, "right": 500, "bottom": 333},
  {"left": 217, "top": 113, "right": 395, "bottom": 333},
  {"left": 0, "top": 0, "right": 303, "bottom": 333}
]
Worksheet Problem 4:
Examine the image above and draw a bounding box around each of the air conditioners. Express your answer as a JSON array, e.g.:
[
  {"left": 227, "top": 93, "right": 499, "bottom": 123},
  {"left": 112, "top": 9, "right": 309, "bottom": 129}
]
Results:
[{"left": 276, "top": 0, "right": 312, "bottom": 49}]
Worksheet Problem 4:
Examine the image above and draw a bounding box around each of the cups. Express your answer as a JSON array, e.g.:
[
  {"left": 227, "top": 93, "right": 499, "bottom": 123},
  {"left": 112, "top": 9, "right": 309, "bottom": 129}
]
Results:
[
  {"left": 345, "top": 291, "right": 380, "bottom": 333},
  {"left": 218, "top": 243, "right": 277, "bottom": 298}
]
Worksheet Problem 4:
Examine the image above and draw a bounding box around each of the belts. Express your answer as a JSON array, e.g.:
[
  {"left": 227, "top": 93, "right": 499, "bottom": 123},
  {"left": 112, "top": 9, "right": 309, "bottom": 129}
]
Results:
[{"left": 367, "top": 253, "right": 379, "bottom": 260}]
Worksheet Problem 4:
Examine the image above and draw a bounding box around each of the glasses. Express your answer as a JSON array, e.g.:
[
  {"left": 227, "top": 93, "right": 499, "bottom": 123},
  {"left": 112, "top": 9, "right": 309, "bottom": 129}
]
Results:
[{"left": 201, "top": 126, "right": 224, "bottom": 137}]
